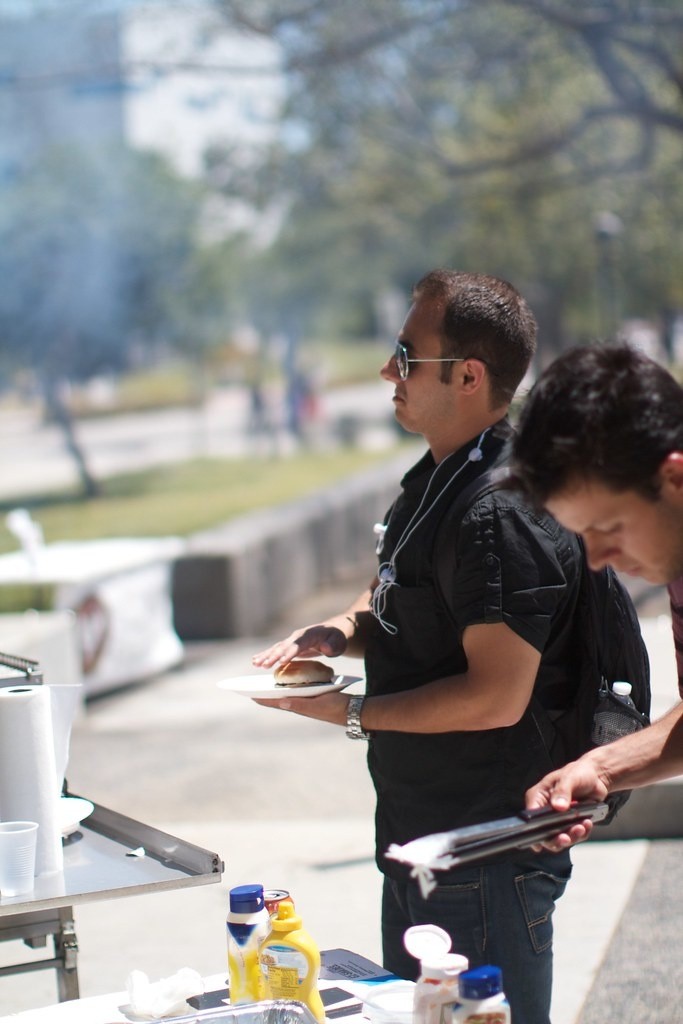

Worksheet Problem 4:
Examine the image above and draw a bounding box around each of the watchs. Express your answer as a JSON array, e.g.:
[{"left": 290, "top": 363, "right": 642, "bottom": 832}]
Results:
[{"left": 346, "top": 695, "right": 370, "bottom": 740}]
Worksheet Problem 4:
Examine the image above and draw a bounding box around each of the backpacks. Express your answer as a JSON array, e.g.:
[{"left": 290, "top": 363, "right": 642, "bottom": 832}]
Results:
[{"left": 432, "top": 465, "right": 651, "bottom": 825}]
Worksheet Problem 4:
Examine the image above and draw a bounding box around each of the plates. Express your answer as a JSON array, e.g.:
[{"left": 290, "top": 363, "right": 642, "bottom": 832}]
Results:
[
  {"left": 216, "top": 675, "right": 365, "bottom": 698},
  {"left": 61, "top": 796, "right": 94, "bottom": 840}
]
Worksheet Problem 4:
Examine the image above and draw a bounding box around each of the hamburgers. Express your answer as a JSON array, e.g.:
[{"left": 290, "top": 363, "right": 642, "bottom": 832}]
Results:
[{"left": 273, "top": 660, "right": 334, "bottom": 688}]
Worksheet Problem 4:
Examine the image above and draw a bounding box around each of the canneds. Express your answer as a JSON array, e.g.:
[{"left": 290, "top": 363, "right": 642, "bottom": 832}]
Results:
[{"left": 264, "top": 890, "right": 295, "bottom": 916}]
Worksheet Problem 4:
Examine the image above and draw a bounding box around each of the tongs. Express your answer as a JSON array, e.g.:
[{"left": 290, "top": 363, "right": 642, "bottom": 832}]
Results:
[{"left": 385, "top": 800, "right": 610, "bottom": 870}]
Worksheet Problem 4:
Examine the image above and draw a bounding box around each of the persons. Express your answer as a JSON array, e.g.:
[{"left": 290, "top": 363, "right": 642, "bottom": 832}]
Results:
[
  {"left": 498, "top": 336, "right": 683, "bottom": 851},
  {"left": 251, "top": 266, "right": 583, "bottom": 1024}
]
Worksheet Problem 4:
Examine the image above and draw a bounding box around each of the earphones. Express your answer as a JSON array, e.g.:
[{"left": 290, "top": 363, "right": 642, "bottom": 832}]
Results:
[
  {"left": 379, "top": 569, "right": 394, "bottom": 582},
  {"left": 373, "top": 524, "right": 387, "bottom": 541}
]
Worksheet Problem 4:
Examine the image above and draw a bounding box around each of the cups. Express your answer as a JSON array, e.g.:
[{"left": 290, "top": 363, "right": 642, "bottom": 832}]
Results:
[{"left": 0, "top": 821, "right": 39, "bottom": 897}]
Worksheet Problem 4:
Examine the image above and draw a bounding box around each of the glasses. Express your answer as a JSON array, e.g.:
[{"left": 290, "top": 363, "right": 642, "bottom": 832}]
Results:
[{"left": 394, "top": 338, "right": 500, "bottom": 382}]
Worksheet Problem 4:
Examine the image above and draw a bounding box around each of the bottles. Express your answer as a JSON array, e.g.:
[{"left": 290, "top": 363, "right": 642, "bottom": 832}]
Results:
[
  {"left": 225, "top": 884, "right": 325, "bottom": 1024},
  {"left": 403, "top": 924, "right": 511, "bottom": 1024}
]
